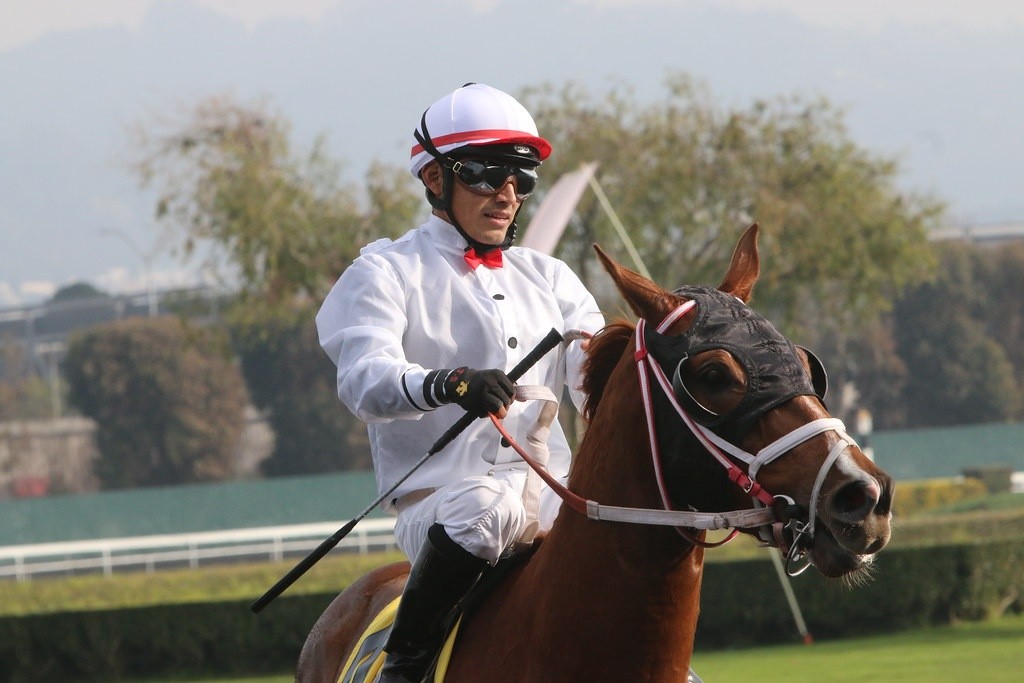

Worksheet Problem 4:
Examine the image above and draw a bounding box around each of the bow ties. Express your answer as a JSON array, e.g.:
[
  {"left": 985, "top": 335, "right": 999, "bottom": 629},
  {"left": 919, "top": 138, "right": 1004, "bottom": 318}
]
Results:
[{"left": 464, "top": 246, "right": 504, "bottom": 270}]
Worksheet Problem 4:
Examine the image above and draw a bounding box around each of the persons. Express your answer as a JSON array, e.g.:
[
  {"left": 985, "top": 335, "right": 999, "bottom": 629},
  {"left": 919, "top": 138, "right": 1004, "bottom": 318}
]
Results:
[{"left": 315, "top": 82, "right": 604, "bottom": 683}]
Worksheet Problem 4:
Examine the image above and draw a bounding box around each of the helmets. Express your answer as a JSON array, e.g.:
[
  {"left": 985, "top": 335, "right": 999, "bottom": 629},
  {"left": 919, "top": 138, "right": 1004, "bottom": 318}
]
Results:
[{"left": 410, "top": 82, "right": 540, "bottom": 177}]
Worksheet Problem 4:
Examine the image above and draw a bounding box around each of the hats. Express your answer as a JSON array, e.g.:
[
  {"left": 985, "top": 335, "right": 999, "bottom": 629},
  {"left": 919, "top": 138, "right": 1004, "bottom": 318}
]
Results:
[{"left": 468, "top": 138, "right": 551, "bottom": 162}]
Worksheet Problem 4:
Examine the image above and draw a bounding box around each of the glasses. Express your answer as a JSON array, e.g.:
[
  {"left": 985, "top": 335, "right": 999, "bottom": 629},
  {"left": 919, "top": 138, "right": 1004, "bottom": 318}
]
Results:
[{"left": 454, "top": 157, "right": 539, "bottom": 200}]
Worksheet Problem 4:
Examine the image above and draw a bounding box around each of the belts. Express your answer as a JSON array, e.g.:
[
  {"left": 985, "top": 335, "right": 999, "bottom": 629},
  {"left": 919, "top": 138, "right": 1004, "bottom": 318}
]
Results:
[{"left": 393, "top": 486, "right": 437, "bottom": 514}]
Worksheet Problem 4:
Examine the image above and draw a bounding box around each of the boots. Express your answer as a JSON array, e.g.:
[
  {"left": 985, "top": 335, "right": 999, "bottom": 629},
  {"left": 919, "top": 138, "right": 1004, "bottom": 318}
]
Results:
[{"left": 376, "top": 524, "right": 477, "bottom": 683}]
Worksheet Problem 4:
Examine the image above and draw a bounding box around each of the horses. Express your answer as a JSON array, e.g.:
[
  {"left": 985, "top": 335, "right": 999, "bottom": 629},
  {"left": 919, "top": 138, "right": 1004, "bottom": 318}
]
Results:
[{"left": 294, "top": 222, "right": 894, "bottom": 683}]
